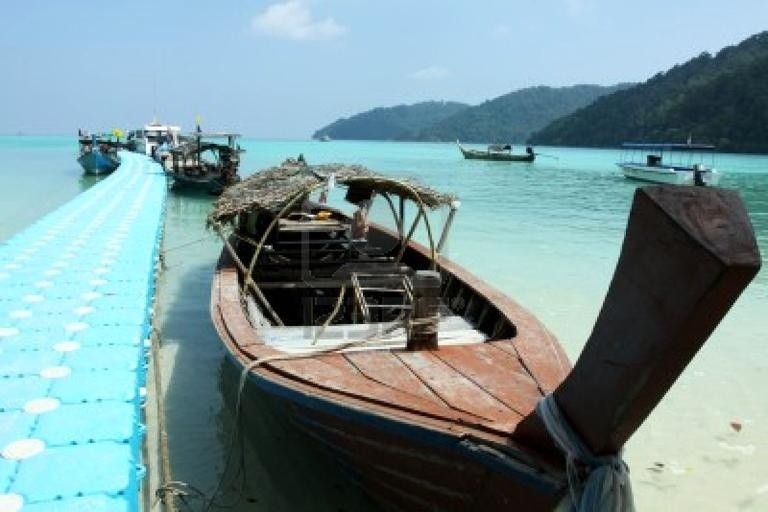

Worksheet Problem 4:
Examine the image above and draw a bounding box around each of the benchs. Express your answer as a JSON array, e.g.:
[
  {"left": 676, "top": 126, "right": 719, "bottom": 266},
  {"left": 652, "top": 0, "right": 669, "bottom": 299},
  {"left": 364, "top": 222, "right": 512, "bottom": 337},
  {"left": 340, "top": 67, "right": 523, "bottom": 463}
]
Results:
[{"left": 256, "top": 238, "right": 391, "bottom": 289}]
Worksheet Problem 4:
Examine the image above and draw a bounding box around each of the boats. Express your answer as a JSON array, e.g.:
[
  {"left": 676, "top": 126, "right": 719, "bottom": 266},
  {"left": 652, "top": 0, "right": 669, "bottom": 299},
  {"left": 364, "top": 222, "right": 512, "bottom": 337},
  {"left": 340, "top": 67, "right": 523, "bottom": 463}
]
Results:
[
  {"left": 210, "top": 156, "right": 762, "bottom": 512},
  {"left": 78, "top": 121, "right": 241, "bottom": 189},
  {"left": 454, "top": 138, "right": 538, "bottom": 165},
  {"left": 608, "top": 140, "right": 721, "bottom": 194}
]
